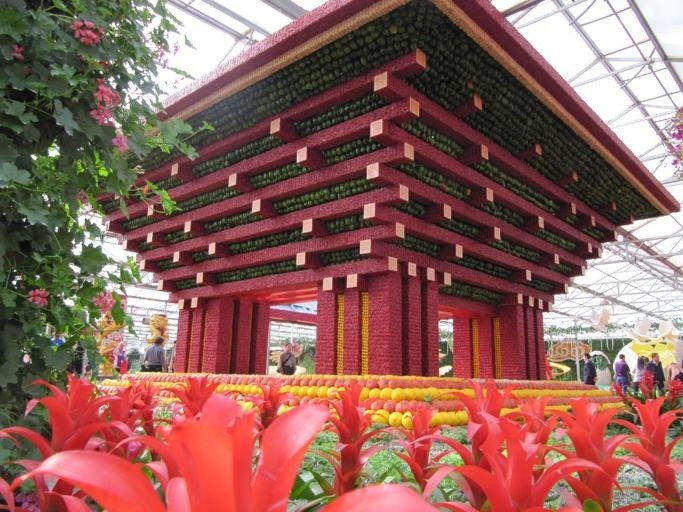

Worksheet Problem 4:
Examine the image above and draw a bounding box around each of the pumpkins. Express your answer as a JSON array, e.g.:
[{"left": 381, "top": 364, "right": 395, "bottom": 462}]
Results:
[{"left": 96, "top": 371, "right": 629, "bottom": 428}]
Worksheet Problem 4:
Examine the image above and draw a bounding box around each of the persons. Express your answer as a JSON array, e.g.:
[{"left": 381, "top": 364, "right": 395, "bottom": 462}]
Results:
[
  {"left": 278, "top": 343, "right": 296, "bottom": 375},
  {"left": 166, "top": 340, "right": 177, "bottom": 372},
  {"left": 71, "top": 341, "right": 83, "bottom": 375},
  {"left": 669, "top": 360, "right": 683, "bottom": 383},
  {"left": 50, "top": 332, "right": 66, "bottom": 347},
  {"left": 614, "top": 354, "right": 630, "bottom": 395},
  {"left": 112, "top": 342, "right": 126, "bottom": 373},
  {"left": 143, "top": 335, "right": 168, "bottom": 373},
  {"left": 632, "top": 352, "right": 666, "bottom": 399},
  {"left": 582, "top": 352, "right": 598, "bottom": 384}
]
